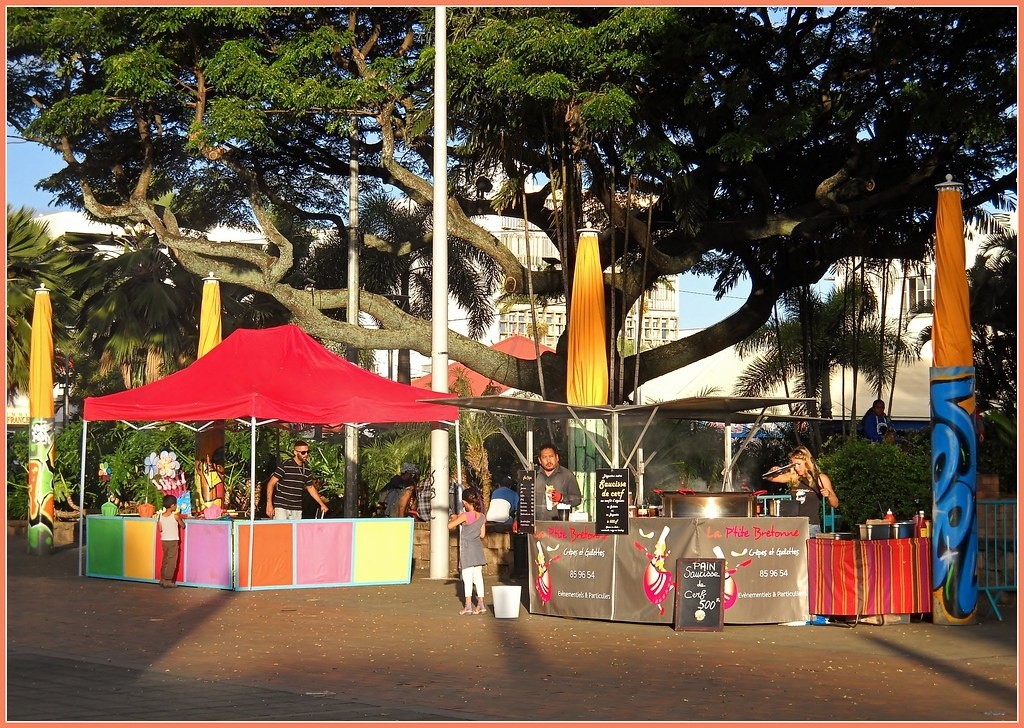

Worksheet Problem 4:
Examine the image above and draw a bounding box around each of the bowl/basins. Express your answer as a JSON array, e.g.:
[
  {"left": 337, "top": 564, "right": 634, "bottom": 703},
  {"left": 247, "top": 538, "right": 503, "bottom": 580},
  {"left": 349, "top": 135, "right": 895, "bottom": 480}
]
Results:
[{"left": 831, "top": 533, "right": 856, "bottom": 540}]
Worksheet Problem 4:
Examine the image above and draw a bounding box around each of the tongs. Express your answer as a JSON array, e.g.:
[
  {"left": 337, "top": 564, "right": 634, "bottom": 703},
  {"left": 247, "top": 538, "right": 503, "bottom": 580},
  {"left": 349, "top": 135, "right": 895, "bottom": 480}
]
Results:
[
  {"left": 806, "top": 456, "right": 824, "bottom": 492},
  {"left": 762, "top": 462, "right": 795, "bottom": 479}
]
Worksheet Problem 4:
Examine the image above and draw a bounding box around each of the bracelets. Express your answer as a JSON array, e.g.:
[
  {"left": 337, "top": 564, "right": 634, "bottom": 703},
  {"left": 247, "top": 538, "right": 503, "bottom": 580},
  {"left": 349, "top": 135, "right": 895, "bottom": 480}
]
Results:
[{"left": 559, "top": 493, "right": 563, "bottom": 502}]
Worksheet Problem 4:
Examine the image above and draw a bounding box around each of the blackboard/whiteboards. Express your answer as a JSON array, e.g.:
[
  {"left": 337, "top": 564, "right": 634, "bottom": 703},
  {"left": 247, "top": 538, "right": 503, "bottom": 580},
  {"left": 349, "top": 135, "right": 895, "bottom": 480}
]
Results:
[{"left": 674, "top": 557, "right": 726, "bottom": 632}]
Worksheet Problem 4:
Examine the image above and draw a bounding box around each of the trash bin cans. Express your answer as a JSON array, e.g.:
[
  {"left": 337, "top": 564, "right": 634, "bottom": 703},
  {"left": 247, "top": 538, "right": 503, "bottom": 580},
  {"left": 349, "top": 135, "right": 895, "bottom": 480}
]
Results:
[{"left": 491, "top": 585, "right": 522, "bottom": 619}]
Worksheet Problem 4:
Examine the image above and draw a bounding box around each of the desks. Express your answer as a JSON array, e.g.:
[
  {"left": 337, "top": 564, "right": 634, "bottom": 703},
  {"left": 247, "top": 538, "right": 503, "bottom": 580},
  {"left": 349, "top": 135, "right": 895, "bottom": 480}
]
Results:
[
  {"left": 86, "top": 515, "right": 414, "bottom": 592},
  {"left": 806, "top": 537, "right": 931, "bottom": 624}
]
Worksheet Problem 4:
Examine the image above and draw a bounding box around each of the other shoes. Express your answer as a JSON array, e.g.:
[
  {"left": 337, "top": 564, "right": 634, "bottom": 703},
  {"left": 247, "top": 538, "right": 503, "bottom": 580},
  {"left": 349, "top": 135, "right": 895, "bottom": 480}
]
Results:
[{"left": 159, "top": 581, "right": 178, "bottom": 589}]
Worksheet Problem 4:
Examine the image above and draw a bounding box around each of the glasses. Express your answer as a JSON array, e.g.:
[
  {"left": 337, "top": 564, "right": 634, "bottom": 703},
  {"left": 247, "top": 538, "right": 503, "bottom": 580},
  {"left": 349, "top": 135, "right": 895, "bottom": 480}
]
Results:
[
  {"left": 296, "top": 449, "right": 312, "bottom": 456},
  {"left": 790, "top": 448, "right": 807, "bottom": 456}
]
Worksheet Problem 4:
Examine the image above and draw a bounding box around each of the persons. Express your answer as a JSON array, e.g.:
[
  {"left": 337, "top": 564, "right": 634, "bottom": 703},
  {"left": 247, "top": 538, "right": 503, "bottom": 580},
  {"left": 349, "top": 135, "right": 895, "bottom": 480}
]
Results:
[
  {"left": 535, "top": 445, "right": 582, "bottom": 521},
  {"left": 266, "top": 441, "right": 328, "bottom": 521},
  {"left": 767, "top": 446, "right": 838, "bottom": 538},
  {"left": 447, "top": 488, "right": 487, "bottom": 616},
  {"left": 487, "top": 478, "right": 520, "bottom": 533},
  {"left": 861, "top": 400, "right": 899, "bottom": 444},
  {"left": 380, "top": 463, "right": 420, "bottom": 518},
  {"left": 159, "top": 495, "right": 185, "bottom": 588}
]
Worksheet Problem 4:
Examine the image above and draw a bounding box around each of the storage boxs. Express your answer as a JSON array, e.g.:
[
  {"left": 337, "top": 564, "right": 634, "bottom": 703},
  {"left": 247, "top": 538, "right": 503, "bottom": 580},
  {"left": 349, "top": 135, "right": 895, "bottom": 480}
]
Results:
[
  {"left": 866, "top": 524, "right": 892, "bottom": 540},
  {"left": 893, "top": 523, "right": 916, "bottom": 538}
]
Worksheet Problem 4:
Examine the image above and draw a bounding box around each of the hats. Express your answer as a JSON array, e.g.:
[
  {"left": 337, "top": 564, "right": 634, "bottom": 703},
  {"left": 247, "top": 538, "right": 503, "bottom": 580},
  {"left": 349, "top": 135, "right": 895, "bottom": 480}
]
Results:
[{"left": 400, "top": 462, "right": 416, "bottom": 473}]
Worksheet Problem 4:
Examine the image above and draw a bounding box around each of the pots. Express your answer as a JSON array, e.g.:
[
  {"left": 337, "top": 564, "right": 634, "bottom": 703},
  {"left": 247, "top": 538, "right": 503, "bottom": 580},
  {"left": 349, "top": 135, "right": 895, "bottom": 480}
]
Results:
[{"left": 653, "top": 489, "right": 766, "bottom": 517}]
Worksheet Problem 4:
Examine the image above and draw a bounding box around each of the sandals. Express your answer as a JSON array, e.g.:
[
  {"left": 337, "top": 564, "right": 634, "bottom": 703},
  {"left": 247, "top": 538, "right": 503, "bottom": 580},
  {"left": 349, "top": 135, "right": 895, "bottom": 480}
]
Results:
[
  {"left": 458, "top": 602, "right": 472, "bottom": 616},
  {"left": 472, "top": 600, "right": 487, "bottom": 616}
]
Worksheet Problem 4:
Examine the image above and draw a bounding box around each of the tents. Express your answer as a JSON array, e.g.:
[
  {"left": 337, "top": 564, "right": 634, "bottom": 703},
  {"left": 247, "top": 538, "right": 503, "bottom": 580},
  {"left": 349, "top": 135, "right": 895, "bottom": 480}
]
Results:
[
  {"left": 79, "top": 322, "right": 463, "bottom": 591},
  {"left": 409, "top": 335, "right": 556, "bottom": 412}
]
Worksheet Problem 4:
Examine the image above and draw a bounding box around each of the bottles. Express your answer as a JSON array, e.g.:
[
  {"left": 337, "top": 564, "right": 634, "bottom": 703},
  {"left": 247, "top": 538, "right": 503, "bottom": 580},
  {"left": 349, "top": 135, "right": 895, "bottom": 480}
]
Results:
[
  {"left": 917, "top": 511, "right": 927, "bottom": 537},
  {"left": 884, "top": 508, "right": 894, "bottom": 523}
]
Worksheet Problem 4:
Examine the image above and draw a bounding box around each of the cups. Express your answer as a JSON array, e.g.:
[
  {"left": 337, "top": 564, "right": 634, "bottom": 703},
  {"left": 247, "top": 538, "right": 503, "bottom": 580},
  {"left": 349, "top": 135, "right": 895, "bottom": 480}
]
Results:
[{"left": 558, "top": 509, "right": 569, "bottom": 521}]
